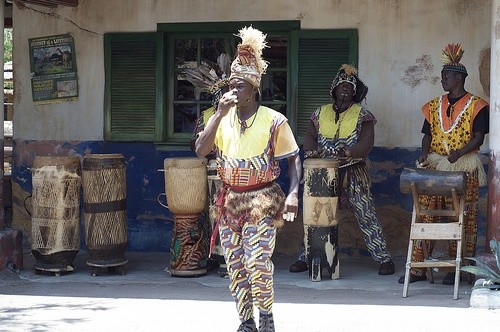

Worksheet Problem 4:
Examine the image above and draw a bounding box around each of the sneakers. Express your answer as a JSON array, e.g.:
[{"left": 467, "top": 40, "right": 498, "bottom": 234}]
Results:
[
  {"left": 258, "top": 313, "right": 275, "bottom": 332},
  {"left": 236, "top": 318, "right": 258, "bottom": 332}
]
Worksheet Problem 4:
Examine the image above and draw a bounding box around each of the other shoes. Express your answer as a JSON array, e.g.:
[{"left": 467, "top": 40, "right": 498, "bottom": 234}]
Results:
[
  {"left": 289, "top": 260, "right": 308, "bottom": 272},
  {"left": 378, "top": 261, "right": 395, "bottom": 275},
  {"left": 443, "top": 272, "right": 462, "bottom": 284},
  {"left": 398, "top": 273, "right": 426, "bottom": 283}
]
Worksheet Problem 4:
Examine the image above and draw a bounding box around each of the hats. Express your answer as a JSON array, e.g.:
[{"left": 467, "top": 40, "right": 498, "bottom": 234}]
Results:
[
  {"left": 440, "top": 43, "right": 469, "bottom": 74},
  {"left": 329, "top": 64, "right": 358, "bottom": 102},
  {"left": 228, "top": 23, "right": 270, "bottom": 88}
]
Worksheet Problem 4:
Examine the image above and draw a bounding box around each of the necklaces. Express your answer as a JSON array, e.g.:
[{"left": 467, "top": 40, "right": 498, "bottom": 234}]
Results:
[
  {"left": 236, "top": 105, "right": 258, "bottom": 133},
  {"left": 333, "top": 101, "right": 353, "bottom": 140}
]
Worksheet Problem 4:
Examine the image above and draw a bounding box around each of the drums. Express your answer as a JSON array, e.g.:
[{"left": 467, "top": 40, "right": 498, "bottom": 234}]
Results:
[
  {"left": 302, "top": 157, "right": 340, "bottom": 282},
  {"left": 208, "top": 161, "right": 228, "bottom": 266},
  {"left": 164, "top": 157, "right": 209, "bottom": 276},
  {"left": 399, "top": 166, "right": 470, "bottom": 196},
  {"left": 29, "top": 155, "right": 80, "bottom": 273},
  {"left": 81, "top": 153, "right": 130, "bottom": 267}
]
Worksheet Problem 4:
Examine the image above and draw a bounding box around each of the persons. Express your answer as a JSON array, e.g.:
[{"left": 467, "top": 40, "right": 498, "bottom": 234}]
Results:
[
  {"left": 289, "top": 65, "right": 395, "bottom": 275},
  {"left": 178, "top": 55, "right": 235, "bottom": 269},
  {"left": 398, "top": 43, "right": 489, "bottom": 285},
  {"left": 195, "top": 27, "right": 301, "bottom": 332}
]
